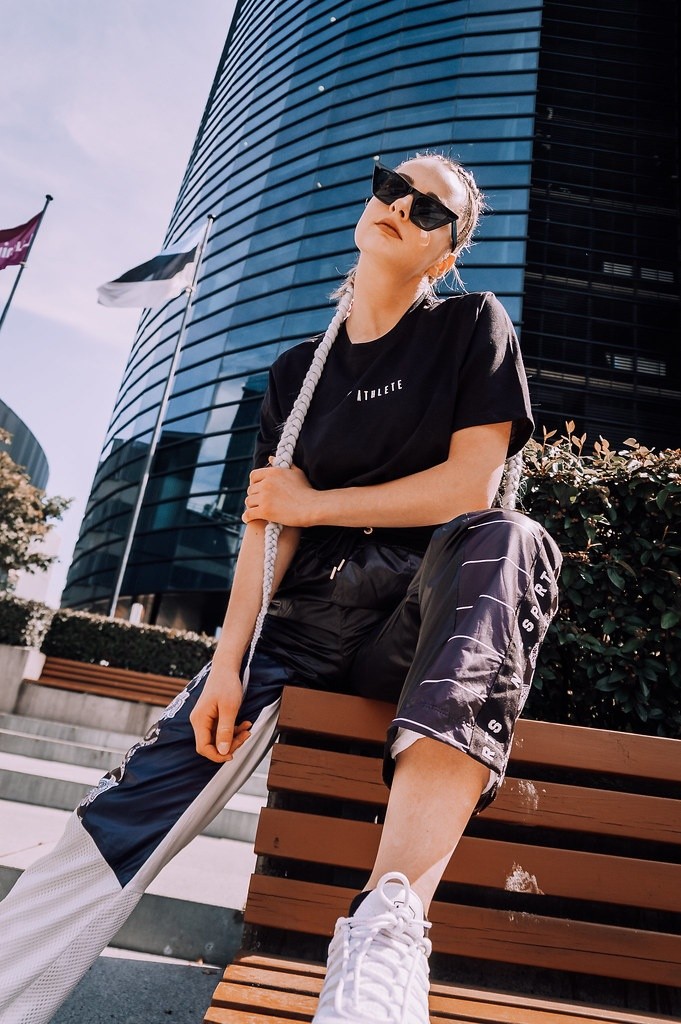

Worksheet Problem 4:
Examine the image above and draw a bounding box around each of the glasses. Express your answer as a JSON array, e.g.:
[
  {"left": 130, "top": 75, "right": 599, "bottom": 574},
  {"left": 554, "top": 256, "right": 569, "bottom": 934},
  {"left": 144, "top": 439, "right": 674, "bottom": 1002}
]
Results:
[{"left": 370, "top": 161, "right": 459, "bottom": 253}]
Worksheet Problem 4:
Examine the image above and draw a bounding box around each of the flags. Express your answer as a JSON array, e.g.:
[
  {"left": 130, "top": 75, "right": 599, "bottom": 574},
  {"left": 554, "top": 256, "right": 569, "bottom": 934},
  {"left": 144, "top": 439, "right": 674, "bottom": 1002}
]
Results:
[
  {"left": 0, "top": 210, "right": 44, "bottom": 269},
  {"left": 97, "top": 219, "right": 210, "bottom": 308}
]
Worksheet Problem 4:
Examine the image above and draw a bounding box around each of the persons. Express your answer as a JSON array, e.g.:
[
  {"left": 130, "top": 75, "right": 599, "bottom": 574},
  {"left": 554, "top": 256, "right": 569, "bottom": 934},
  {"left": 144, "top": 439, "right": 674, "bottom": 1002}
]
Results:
[{"left": 0, "top": 154, "right": 563, "bottom": 1024}]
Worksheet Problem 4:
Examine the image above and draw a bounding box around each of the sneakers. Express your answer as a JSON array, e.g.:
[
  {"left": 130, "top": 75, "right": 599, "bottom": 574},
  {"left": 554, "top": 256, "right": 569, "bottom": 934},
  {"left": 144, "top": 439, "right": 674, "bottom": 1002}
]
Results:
[{"left": 309, "top": 871, "right": 433, "bottom": 1024}]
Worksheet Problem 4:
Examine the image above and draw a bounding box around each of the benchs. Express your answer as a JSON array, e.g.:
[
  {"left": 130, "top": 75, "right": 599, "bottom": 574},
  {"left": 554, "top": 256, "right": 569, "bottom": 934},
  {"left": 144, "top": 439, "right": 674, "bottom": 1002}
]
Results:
[
  {"left": 201, "top": 685, "right": 681, "bottom": 1024},
  {"left": 24, "top": 657, "right": 193, "bottom": 707}
]
